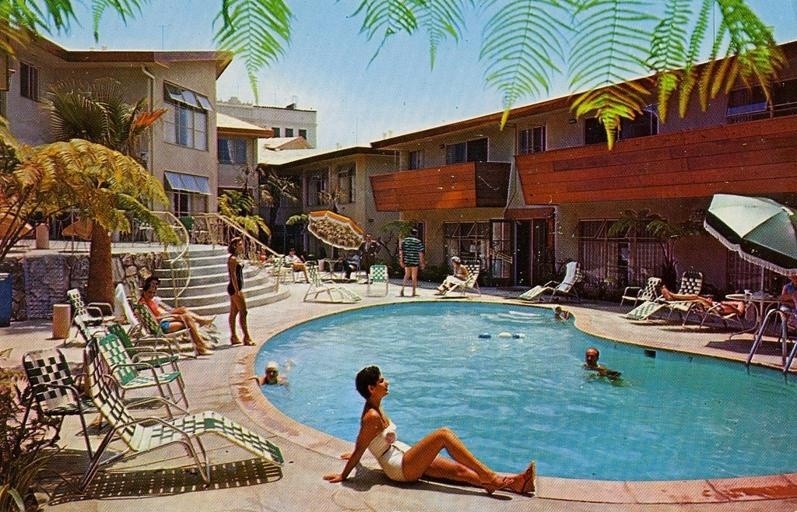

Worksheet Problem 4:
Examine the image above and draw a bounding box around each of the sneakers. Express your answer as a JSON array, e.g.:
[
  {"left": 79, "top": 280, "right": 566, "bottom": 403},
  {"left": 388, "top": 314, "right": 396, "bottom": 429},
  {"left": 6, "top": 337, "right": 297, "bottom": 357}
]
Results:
[
  {"left": 400, "top": 287, "right": 404, "bottom": 296},
  {"left": 443, "top": 284, "right": 449, "bottom": 290},
  {"left": 197, "top": 314, "right": 217, "bottom": 355}
]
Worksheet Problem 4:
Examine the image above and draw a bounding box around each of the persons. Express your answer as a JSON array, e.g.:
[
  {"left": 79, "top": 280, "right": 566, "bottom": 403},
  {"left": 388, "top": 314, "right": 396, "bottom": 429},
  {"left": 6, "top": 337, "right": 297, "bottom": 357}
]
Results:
[
  {"left": 225, "top": 236, "right": 256, "bottom": 346},
  {"left": 284, "top": 248, "right": 310, "bottom": 283},
  {"left": 320, "top": 365, "right": 535, "bottom": 497},
  {"left": 583, "top": 347, "right": 621, "bottom": 381},
  {"left": 658, "top": 283, "right": 745, "bottom": 317},
  {"left": 779, "top": 268, "right": 796, "bottom": 331},
  {"left": 144, "top": 276, "right": 217, "bottom": 340},
  {"left": 435, "top": 256, "right": 468, "bottom": 293},
  {"left": 136, "top": 282, "right": 213, "bottom": 355},
  {"left": 553, "top": 307, "right": 569, "bottom": 320},
  {"left": 358, "top": 232, "right": 381, "bottom": 284},
  {"left": 397, "top": 228, "right": 426, "bottom": 296},
  {"left": 341, "top": 249, "right": 360, "bottom": 282},
  {"left": 247, "top": 360, "right": 289, "bottom": 387}
]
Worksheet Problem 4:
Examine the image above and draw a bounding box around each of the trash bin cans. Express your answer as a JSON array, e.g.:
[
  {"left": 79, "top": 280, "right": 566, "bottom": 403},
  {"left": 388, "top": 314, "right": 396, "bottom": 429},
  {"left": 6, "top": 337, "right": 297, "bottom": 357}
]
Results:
[
  {"left": 0, "top": 272, "right": 12, "bottom": 328},
  {"left": 52, "top": 303, "right": 71, "bottom": 339}
]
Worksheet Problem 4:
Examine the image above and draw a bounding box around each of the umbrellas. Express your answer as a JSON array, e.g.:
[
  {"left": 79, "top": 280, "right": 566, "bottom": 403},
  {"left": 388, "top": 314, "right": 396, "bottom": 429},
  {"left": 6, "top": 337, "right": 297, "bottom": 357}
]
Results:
[
  {"left": 306, "top": 210, "right": 365, "bottom": 257},
  {"left": 701, "top": 190, "right": 797, "bottom": 321}
]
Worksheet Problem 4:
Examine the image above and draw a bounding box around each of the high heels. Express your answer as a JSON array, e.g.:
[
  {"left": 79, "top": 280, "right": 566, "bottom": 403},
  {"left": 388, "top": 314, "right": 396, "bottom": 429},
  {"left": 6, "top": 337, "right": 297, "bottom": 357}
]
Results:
[
  {"left": 521, "top": 460, "right": 535, "bottom": 493},
  {"left": 480, "top": 473, "right": 515, "bottom": 494},
  {"left": 231, "top": 335, "right": 256, "bottom": 345}
]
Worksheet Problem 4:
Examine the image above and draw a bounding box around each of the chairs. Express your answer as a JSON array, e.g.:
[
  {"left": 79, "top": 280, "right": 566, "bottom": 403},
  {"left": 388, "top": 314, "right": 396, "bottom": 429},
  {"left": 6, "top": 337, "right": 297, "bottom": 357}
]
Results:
[
  {"left": 519, "top": 260, "right": 581, "bottom": 304},
  {"left": 617, "top": 270, "right": 753, "bottom": 333},
  {"left": 275, "top": 254, "right": 389, "bottom": 304},
  {"left": 441, "top": 264, "right": 481, "bottom": 297}
]
